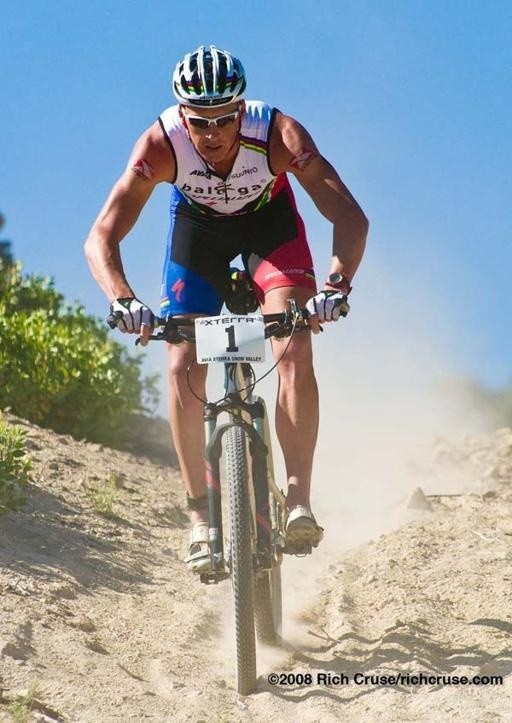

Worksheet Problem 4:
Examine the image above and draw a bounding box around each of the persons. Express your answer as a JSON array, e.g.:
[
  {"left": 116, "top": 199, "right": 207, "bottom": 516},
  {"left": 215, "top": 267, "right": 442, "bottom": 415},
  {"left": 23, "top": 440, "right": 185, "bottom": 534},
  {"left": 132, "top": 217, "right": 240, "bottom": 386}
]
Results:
[{"left": 82, "top": 40, "right": 373, "bottom": 576}]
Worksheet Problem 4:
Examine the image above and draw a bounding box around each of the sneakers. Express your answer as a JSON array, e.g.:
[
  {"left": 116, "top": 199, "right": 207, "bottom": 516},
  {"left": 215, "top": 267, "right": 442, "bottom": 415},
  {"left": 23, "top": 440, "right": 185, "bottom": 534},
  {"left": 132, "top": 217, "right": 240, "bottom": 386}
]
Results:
[
  {"left": 186, "top": 520, "right": 212, "bottom": 573},
  {"left": 284, "top": 505, "right": 321, "bottom": 549}
]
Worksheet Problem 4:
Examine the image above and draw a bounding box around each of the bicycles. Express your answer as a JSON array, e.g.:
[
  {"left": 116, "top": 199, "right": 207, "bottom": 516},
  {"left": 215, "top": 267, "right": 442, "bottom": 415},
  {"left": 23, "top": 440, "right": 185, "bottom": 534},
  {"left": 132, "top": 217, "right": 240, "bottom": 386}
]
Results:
[{"left": 108, "top": 301, "right": 351, "bottom": 693}]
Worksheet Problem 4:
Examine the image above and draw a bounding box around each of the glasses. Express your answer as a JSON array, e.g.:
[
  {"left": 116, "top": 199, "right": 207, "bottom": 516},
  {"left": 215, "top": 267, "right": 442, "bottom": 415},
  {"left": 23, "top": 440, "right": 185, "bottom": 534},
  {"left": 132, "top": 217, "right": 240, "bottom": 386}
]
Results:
[{"left": 181, "top": 104, "right": 242, "bottom": 129}]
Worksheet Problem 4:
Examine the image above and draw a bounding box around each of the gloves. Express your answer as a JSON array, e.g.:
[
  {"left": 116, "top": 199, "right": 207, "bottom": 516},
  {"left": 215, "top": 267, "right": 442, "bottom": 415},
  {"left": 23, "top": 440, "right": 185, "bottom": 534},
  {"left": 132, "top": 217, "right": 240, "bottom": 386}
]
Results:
[
  {"left": 304, "top": 288, "right": 348, "bottom": 322},
  {"left": 109, "top": 297, "right": 156, "bottom": 335}
]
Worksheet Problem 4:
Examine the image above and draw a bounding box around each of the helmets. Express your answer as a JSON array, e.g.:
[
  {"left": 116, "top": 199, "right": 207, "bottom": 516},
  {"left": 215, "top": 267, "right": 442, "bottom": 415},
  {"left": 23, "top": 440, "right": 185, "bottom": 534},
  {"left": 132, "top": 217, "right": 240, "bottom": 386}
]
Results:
[{"left": 172, "top": 44, "right": 248, "bottom": 109}]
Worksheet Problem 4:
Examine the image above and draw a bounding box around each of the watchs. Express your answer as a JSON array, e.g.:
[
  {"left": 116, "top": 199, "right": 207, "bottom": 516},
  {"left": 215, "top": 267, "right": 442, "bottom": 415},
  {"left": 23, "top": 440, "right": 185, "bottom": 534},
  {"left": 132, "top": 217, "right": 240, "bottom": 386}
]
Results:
[{"left": 322, "top": 269, "right": 354, "bottom": 295}]
